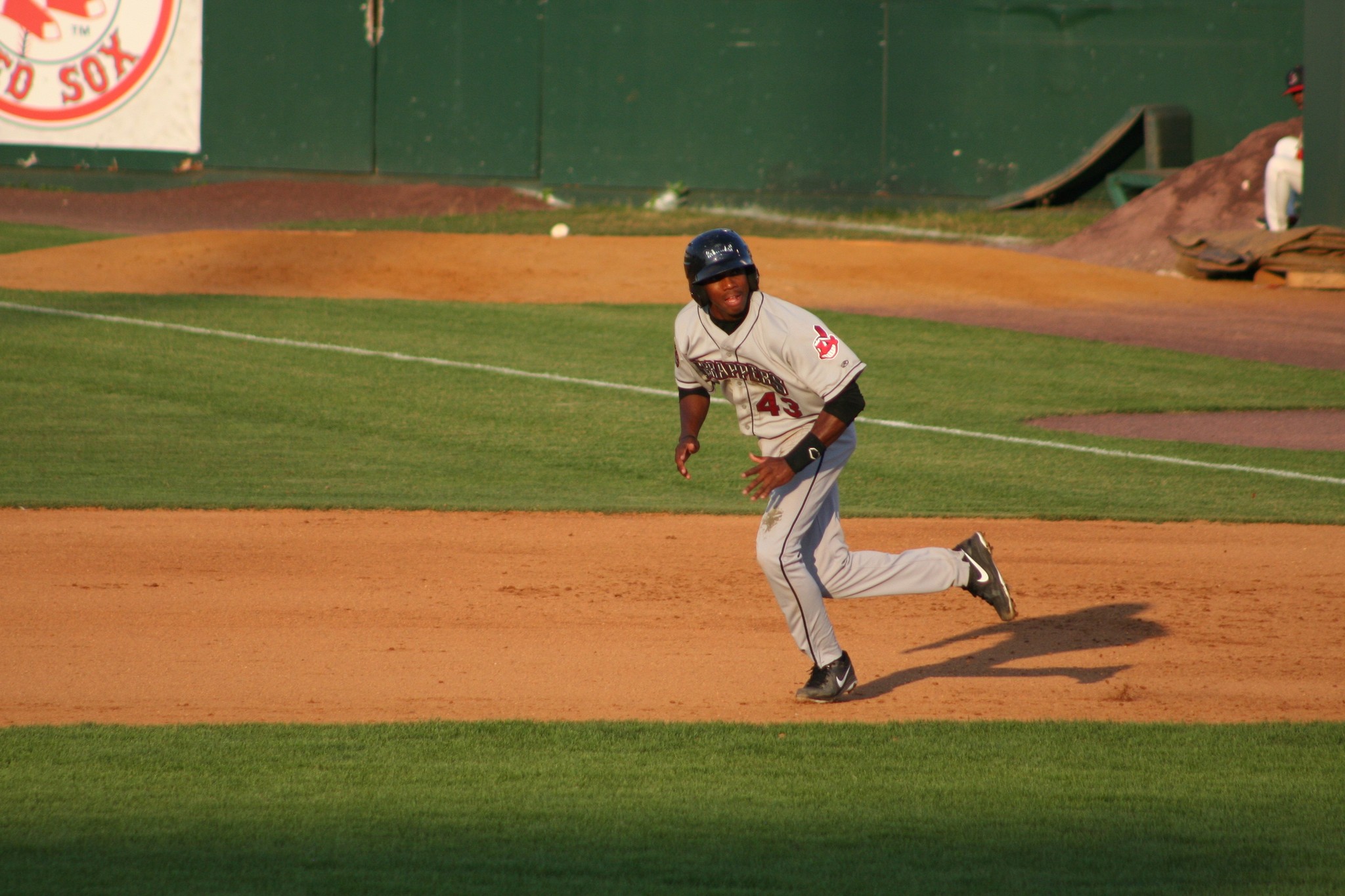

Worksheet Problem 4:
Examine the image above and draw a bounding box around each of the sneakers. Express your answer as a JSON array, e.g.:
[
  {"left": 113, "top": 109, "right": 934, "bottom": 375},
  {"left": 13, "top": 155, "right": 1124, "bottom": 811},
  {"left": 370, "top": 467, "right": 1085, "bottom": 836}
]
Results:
[
  {"left": 957, "top": 532, "right": 1016, "bottom": 620},
  {"left": 796, "top": 648, "right": 856, "bottom": 703}
]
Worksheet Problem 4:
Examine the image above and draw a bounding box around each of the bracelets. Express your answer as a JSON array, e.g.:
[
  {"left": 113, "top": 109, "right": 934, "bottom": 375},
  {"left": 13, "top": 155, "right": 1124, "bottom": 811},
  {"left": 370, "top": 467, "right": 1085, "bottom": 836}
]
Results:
[{"left": 784, "top": 432, "right": 826, "bottom": 474}]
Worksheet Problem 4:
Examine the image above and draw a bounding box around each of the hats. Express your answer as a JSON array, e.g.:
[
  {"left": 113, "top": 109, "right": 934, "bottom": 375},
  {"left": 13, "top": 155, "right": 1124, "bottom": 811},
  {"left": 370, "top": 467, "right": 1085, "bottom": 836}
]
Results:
[{"left": 1283, "top": 65, "right": 1304, "bottom": 95}]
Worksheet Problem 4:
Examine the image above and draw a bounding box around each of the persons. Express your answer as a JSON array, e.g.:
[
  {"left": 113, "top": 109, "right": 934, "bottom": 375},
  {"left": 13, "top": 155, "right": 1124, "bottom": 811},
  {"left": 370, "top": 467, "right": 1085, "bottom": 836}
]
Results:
[
  {"left": 673, "top": 228, "right": 1016, "bottom": 703},
  {"left": 1265, "top": 66, "right": 1304, "bottom": 231}
]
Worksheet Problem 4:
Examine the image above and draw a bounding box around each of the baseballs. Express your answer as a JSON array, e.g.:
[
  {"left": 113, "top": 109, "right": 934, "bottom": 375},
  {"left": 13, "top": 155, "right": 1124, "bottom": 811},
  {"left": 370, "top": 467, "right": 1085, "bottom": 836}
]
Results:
[{"left": 550, "top": 223, "right": 570, "bottom": 238}]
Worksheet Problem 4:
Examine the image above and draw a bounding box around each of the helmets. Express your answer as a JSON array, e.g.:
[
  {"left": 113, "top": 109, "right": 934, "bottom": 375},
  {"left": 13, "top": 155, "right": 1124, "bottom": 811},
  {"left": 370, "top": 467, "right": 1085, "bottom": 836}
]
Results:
[{"left": 684, "top": 226, "right": 760, "bottom": 306}]
